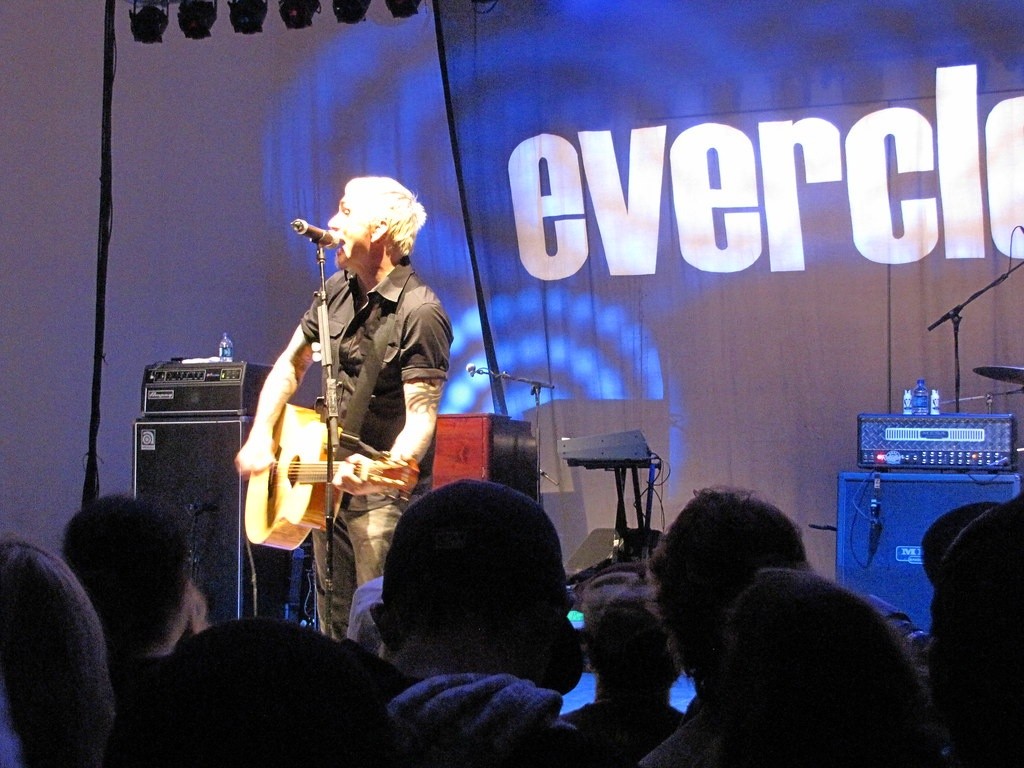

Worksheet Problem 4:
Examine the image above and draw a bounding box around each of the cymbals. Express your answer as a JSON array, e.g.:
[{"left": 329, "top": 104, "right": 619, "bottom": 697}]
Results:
[{"left": 973, "top": 366, "right": 1024, "bottom": 385}]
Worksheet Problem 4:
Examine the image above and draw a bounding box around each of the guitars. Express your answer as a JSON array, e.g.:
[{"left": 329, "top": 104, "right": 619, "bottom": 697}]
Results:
[{"left": 245, "top": 403, "right": 418, "bottom": 553}]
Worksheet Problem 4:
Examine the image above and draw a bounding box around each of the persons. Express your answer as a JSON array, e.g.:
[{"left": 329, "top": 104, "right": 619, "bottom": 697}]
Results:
[
  {"left": 1, "top": 480, "right": 1024, "bottom": 768},
  {"left": 235, "top": 177, "right": 456, "bottom": 641}
]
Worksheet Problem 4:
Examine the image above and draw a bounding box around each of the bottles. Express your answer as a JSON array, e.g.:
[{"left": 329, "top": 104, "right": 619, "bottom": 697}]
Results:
[
  {"left": 913, "top": 380, "right": 929, "bottom": 416},
  {"left": 903, "top": 390, "right": 912, "bottom": 414},
  {"left": 219, "top": 333, "right": 233, "bottom": 362},
  {"left": 931, "top": 389, "right": 939, "bottom": 415}
]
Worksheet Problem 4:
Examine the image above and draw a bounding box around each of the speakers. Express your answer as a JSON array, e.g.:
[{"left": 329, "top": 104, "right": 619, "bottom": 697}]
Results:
[
  {"left": 836, "top": 471, "right": 1022, "bottom": 636},
  {"left": 132, "top": 416, "right": 315, "bottom": 633},
  {"left": 564, "top": 527, "right": 663, "bottom": 580}
]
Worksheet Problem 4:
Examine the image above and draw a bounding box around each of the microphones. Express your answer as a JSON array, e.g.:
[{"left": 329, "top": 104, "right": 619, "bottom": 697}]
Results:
[
  {"left": 870, "top": 500, "right": 879, "bottom": 529},
  {"left": 466, "top": 363, "right": 476, "bottom": 377},
  {"left": 290, "top": 218, "right": 340, "bottom": 249},
  {"left": 195, "top": 503, "right": 217, "bottom": 511}
]
